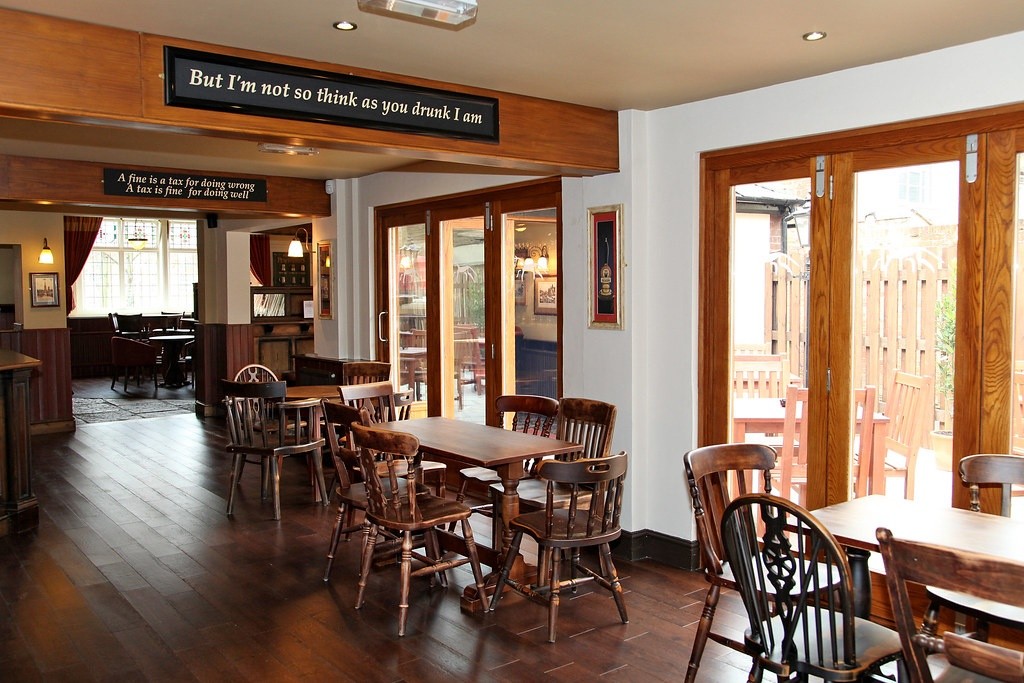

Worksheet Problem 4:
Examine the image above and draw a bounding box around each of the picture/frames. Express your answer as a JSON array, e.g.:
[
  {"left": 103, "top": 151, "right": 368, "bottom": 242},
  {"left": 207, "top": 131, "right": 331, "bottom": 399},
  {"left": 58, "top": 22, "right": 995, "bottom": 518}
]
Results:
[
  {"left": 515, "top": 277, "right": 526, "bottom": 306},
  {"left": 588, "top": 203, "right": 623, "bottom": 329},
  {"left": 535, "top": 273, "right": 557, "bottom": 315},
  {"left": 317, "top": 241, "right": 333, "bottom": 319},
  {"left": 29, "top": 271, "right": 60, "bottom": 307}
]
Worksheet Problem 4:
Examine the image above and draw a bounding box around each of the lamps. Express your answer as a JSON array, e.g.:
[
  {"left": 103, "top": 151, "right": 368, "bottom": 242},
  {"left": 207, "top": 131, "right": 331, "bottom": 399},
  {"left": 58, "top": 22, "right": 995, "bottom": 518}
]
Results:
[
  {"left": 37, "top": 238, "right": 54, "bottom": 265},
  {"left": 128, "top": 219, "right": 147, "bottom": 250},
  {"left": 356, "top": 0, "right": 478, "bottom": 32},
  {"left": 514, "top": 244, "right": 549, "bottom": 281},
  {"left": 287, "top": 228, "right": 310, "bottom": 257},
  {"left": 257, "top": 142, "right": 320, "bottom": 157}
]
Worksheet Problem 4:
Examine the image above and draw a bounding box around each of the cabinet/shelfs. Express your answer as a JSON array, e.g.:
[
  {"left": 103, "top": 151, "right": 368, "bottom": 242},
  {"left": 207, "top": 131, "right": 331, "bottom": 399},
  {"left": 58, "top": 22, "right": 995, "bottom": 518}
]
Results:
[{"left": 252, "top": 286, "right": 314, "bottom": 371}]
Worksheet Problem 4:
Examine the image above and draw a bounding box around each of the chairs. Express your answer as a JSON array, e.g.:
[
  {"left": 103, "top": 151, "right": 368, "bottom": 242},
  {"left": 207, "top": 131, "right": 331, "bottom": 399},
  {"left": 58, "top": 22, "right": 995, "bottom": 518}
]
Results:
[{"left": 108, "top": 311, "right": 1024, "bottom": 683}]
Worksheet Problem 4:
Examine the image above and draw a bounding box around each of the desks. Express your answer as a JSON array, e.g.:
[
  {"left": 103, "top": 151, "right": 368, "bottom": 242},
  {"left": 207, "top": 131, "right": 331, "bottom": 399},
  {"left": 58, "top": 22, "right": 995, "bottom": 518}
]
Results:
[
  {"left": 785, "top": 495, "right": 1024, "bottom": 620},
  {"left": 370, "top": 417, "right": 584, "bottom": 613},
  {"left": 286, "top": 385, "right": 343, "bottom": 484},
  {"left": 149, "top": 334, "right": 196, "bottom": 386},
  {"left": 459, "top": 337, "right": 486, "bottom": 396},
  {"left": 733, "top": 397, "right": 890, "bottom": 528},
  {"left": 400, "top": 347, "right": 427, "bottom": 400},
  {"left": 0, "top": 348, "right": 43, "bottom": 537}
]
